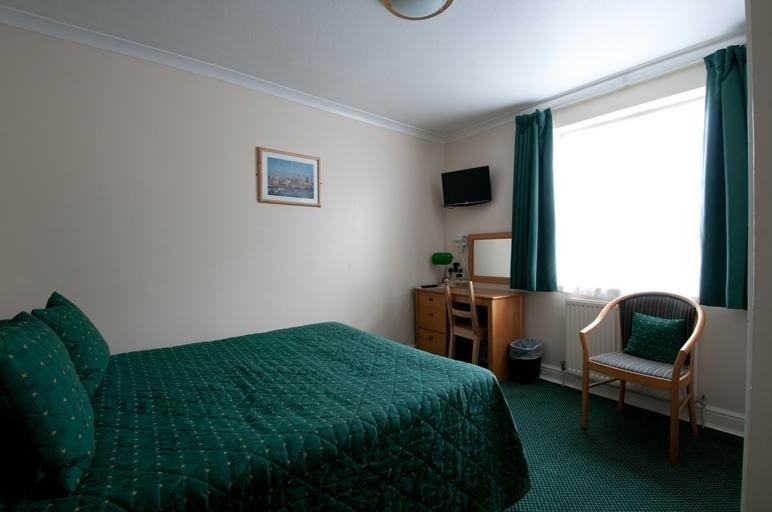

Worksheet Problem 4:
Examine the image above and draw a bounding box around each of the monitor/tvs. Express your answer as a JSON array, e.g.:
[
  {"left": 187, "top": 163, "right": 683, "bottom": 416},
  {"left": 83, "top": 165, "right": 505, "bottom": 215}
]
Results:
[{"left": 441, "top": 165, "right": 491, "bottom": 208}]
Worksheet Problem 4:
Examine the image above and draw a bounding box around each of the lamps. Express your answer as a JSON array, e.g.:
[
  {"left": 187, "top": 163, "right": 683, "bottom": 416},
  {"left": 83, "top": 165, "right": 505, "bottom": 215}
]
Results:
[
  {"left": 432, "top": 252, "right": 452, "bottom": 283},
  {"left": 384, "top": 0, "right": 455, "bottom": 20}
]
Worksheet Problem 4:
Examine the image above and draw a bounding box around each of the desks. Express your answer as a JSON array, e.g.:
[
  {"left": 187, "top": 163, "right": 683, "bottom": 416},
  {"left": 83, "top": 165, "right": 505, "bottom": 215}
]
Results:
[{"left": 414, "top": 287, "right": 525, "bottom": 384}]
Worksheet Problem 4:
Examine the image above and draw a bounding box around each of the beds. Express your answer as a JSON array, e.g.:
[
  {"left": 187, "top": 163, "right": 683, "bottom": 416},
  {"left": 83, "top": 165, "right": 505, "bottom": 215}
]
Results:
[{"left": 0, "top": 322, "right": 531, "bottom": 512}]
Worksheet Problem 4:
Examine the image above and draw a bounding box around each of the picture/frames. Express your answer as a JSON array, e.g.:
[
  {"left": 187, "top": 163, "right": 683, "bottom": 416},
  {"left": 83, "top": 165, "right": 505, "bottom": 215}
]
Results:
[{"left": 256, "top": 146, "right": 321, "bottom": 207}]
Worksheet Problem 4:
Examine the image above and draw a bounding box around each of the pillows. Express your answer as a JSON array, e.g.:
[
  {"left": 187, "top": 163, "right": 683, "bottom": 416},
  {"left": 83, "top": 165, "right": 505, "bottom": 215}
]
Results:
[
  {"left": 624, "top": 311, "right": 685, "bottom": 363},
  {"left": 31, "top": 290, "right": 110, "bottom": 399},
  {"left": 0, "top": 311, "right": 96, "bottom": 494}
]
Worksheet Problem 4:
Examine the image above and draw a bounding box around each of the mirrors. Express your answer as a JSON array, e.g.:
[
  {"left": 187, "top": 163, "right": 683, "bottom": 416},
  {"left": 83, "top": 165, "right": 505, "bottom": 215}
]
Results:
[{"left": 468, "top": 232, "right": 512, "bottom": 284}]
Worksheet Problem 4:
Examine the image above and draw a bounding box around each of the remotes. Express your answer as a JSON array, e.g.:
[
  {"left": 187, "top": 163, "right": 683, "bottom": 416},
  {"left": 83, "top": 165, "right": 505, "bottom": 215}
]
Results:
[{"left": 422, "top": 285, "right": 438, "bottom": 288}]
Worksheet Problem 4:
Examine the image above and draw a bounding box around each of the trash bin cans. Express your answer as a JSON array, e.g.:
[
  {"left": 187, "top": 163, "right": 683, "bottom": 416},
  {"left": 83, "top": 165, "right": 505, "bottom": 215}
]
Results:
[{"left": 509, "top": 336, "right": 545, "bottom": 385}]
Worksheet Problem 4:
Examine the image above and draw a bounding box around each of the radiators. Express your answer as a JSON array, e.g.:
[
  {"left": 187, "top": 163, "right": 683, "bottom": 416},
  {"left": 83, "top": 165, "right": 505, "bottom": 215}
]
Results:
[{"left": 560, "top": 298, "right": 706, "bottom": 428}]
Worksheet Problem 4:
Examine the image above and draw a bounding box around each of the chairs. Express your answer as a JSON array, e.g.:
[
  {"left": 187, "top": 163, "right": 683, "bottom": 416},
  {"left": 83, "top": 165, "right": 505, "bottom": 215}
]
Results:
[
  {"left": 443, "top": 279, "right": 488, "bottom": 366},
  {"left": 578, "top": 292, "right": 704, "bottom": 464}
]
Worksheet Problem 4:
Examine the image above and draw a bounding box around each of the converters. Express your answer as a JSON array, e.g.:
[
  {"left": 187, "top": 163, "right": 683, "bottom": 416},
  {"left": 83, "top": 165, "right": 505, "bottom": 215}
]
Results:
[{"left": 453, "top": 263, "right": 460, "bottom": 272}]
